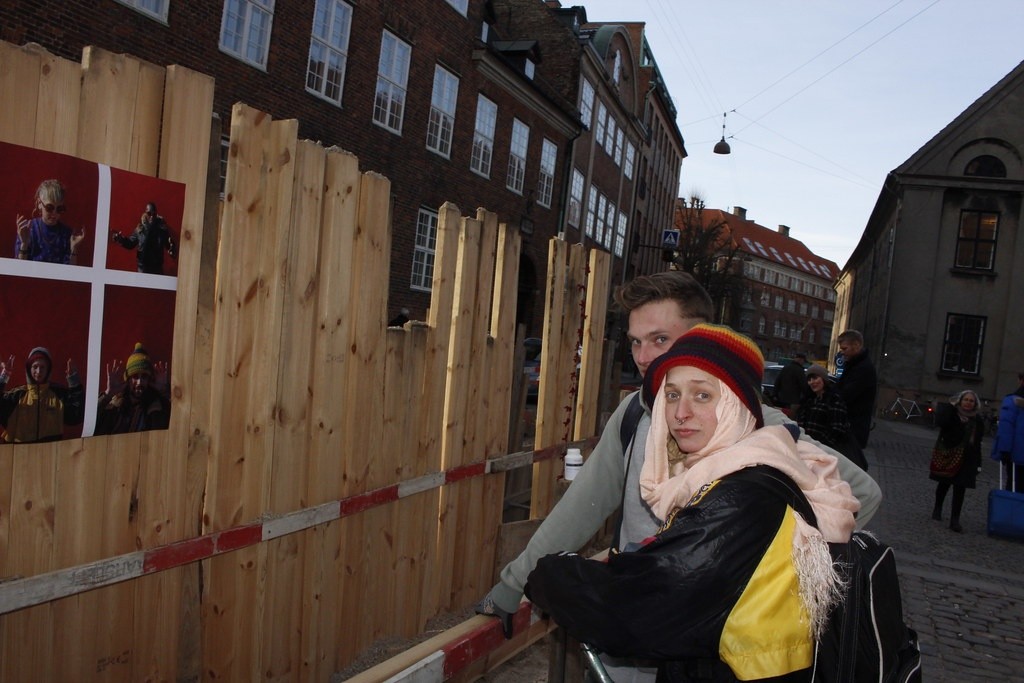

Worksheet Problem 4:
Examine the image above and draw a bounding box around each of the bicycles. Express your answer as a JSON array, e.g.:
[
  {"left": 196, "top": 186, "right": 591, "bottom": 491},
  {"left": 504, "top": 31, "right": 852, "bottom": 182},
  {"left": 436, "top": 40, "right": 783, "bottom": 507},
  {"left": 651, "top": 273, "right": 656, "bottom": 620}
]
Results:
[{"left": 883, "top": 390, "right": 928, "bottom": 424}]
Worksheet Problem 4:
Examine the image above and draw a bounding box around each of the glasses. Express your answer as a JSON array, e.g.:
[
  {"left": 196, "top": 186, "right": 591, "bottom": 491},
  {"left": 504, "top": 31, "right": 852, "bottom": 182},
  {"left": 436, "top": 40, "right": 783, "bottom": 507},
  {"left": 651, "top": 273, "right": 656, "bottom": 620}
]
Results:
[
  {"left": 40, "top": 200, "right": 65, "bottom": 214},
  {"left": 146, "top": 211, "right": 156, "bottom": 216}
]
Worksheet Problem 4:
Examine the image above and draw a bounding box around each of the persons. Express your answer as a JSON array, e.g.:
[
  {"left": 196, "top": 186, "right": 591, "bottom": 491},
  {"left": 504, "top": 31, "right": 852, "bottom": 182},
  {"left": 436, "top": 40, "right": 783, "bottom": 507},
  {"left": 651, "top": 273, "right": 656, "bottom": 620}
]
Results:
[
  {"left": 14, "top": 178, "right": 87, "bottom": 265},
  {"left": 928, "top": 390, "right": 985, "bottom": 534},
  {"left": 0, "top": 344, "right": 85, "bottom": 444},
  {"left": 992, "top": 380, "right": 1024, "bottom": 495},
  {"left": 474, "top": 272, "right": 884, "bottom": 683},
  {"left": 522, "top": 321, "right": 834, "bottom": 683},
  {"left": 110, "top": 201, "right": 177, "bottom": 275},
  {"left": 794, "top": 364, "right": 868, "bottom": 472},
  {"left": 837, "top": 329, "right": 877, "bottom": 450},
  {"left": 774, "top": 352, "right": 809, "bottom": 410},
  {"left": 94, "top": 343, "right": 171, "bottom": 434}
]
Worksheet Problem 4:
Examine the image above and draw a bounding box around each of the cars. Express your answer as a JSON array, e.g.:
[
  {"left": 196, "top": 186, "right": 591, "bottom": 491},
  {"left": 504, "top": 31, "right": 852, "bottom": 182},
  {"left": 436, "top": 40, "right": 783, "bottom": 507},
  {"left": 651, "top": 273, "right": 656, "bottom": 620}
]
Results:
[{"left": 762, "top": 365, "right": 838, "bottom": 404}]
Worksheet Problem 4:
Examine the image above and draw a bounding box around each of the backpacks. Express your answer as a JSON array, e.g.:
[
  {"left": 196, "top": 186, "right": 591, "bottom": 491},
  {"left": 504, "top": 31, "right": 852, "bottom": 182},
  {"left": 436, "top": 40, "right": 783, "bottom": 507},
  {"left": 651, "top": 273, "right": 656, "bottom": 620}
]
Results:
[{"left": 720, "top": 464, "right": 922, "bottom": 683}]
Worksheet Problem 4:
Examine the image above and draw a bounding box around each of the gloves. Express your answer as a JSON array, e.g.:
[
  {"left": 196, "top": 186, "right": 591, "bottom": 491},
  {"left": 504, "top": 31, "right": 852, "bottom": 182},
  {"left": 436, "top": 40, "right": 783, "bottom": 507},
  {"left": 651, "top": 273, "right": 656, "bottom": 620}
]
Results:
[{"left": 475, "top": 591, "right": 513, "bottom": 640}]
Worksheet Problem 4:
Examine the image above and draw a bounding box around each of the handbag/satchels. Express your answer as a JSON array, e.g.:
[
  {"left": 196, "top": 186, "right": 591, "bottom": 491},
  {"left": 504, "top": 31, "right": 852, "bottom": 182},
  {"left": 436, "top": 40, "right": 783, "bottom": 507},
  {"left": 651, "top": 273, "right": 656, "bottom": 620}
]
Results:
[{"left": 930, "top": 439, "right": 972, "bottom": 477}]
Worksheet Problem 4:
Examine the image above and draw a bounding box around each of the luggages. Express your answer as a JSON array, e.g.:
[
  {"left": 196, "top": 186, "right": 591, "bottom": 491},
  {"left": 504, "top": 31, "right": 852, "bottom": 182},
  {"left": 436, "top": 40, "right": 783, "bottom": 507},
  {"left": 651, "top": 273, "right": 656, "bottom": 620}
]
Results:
[{"left": 987, "top": 460, "right": 1024, "bottom": 537}]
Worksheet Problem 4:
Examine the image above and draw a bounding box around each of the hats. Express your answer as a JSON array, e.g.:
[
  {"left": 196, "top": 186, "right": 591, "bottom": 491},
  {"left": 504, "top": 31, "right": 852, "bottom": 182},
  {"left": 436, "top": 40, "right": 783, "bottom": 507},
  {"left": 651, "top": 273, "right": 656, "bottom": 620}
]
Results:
[
  {"left": 29, "top": 352, "right": 49, "bottom": 365},
  {"left": 124, "top": 342, "right": 153, "bottom": 382},
  {"left": 794, "top": 352, "right": 808, "bottom": 363},
  {"left": 643, "top": 322, "right": 765, "bottom": 430},
  {"left": 805, "top": 364, "right": 829, "bottom": 381}
]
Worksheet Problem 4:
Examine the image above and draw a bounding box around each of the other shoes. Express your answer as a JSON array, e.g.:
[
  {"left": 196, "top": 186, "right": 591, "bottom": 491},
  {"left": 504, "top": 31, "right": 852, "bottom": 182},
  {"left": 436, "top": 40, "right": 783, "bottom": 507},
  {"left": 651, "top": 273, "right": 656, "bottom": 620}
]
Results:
[
  {"left": 933, "top": 512, "right": 943, "bottom": 521},
  {"left": 949, "top": 524, "right": 967, "bottom": 534}
]
